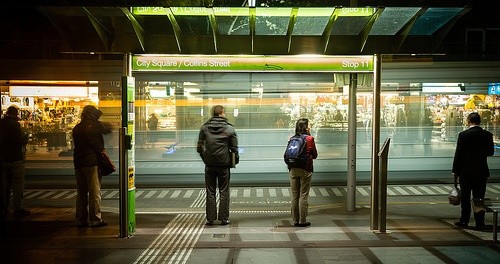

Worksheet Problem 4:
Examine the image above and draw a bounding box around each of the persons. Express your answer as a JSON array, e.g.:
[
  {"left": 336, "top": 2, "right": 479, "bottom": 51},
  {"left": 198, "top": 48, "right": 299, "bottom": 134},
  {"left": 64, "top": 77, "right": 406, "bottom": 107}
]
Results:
[
  {"left": 197, "top": 105, "right": 239, "bottom": 226},
  {"left": 422, "top": 108, "right": 433, "bottom": 156},
  {"left": 0, "top": 106, "right": 31, "bottom": 217},
  {"left": 282, "top": 105, "right": 404, "bottom": 126},
  {"left": 284, "top": 118, "right": 318, "bottom": 227},
  {"left": 146, "top": 113, "right": 158, "bottom": 130},
  {"left": 72, "top": 105, "right": 108, "bottom": 228},
  {"left": 451, "top": 113, "right": 494, "bottom": 229}
]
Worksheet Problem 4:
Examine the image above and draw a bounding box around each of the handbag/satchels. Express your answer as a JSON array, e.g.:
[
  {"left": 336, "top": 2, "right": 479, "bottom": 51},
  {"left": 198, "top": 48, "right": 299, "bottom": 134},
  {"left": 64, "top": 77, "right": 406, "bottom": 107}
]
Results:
[{"left": 448, "top": 175, "right": 461, "bottom": 205}]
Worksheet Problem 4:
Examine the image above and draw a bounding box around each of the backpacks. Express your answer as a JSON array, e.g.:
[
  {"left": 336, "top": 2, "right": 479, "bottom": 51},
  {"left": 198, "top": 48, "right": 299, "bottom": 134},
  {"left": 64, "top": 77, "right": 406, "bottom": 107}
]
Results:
[{"left": 285, "top": 134, "right": 308, "bottom": 160}]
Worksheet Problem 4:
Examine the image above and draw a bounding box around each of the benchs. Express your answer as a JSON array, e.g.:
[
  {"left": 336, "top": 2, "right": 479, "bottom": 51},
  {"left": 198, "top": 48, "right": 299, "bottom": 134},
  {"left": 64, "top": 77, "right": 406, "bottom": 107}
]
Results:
[{"left": 472, "top": 197, "right": 500, "bottom": 244}]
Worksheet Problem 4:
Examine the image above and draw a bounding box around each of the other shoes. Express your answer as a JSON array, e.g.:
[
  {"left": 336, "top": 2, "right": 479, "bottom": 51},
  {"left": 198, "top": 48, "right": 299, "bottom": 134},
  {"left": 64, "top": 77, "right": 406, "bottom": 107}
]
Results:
[
  {"left": 294, "top": 223, "right": 299, "bottom": 226},
  {"left": 93, "top": 221, "right": 108, "bottom": 228},
  {"left": 454, "top": 221, "right": 468, "bottom": 228},
  {"left": 221, "top": 221, "right": 230, "bottom": 225},
  {"left": 299, "top": 222, "right": 311, "bottom": 227},
  {"left": 206, "top": 221, "right": 214, "bottom": 226},
  {"left": 476, "top": 223, "right": 486, "bottom": 229}
]
